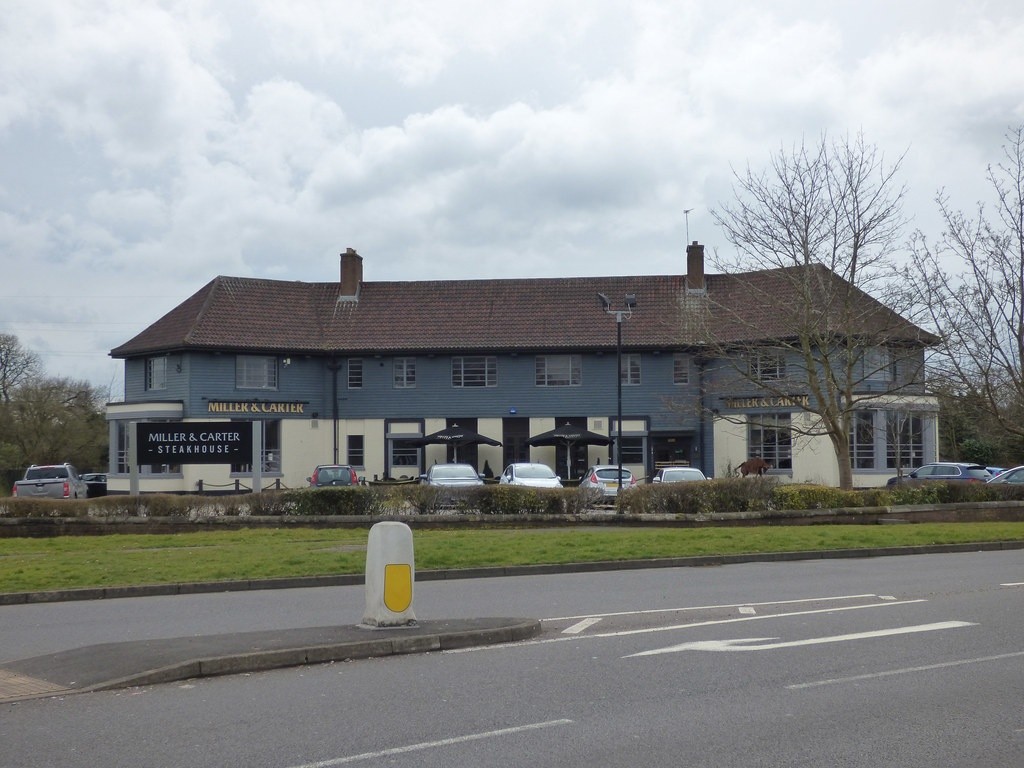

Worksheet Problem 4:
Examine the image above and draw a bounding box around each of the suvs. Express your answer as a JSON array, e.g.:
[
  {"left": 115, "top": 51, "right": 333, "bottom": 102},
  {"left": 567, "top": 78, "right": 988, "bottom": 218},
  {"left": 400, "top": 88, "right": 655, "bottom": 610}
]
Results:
[{"left": 886, "top": 462, "right": 994, "bottom": 490}]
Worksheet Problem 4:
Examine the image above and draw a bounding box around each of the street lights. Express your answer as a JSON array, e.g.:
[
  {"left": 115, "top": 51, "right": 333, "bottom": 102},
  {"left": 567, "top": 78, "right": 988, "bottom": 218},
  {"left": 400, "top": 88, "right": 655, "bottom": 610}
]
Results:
[{"left": 595, "top": 289, "right": 636, "bottom": 518}]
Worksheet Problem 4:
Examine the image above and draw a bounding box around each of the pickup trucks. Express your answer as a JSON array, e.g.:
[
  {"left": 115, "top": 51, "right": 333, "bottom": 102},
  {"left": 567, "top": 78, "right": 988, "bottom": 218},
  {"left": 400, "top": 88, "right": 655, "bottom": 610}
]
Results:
[{"left": 12, "top": 462, "right": 89, "bottom": 503}]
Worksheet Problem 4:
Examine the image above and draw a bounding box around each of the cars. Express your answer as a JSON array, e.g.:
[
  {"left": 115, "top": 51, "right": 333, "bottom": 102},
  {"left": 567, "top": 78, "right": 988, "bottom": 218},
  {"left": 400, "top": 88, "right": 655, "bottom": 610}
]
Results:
[
  {"left": 982, "top": 466, "right": 1024, "bottom": 487},
  {"left": 985, "top": 466, "right": 1019, "bottom": 482},
  {"left": 651, "top": 466, "right": 713, "bottom": 505},
  {"left": 78, "top": 472, "right": 107, "bottom": 498},
  {"left": 306, "top": 464, "right": 360, "bottom": 487},
  {"left": 500, "top": 462, "right": 565, "bottom": 489},
  {"left": 579, "top": 464, "right": 638, "bottom": 509},
  {"left": 419, "top": 463, "right": 491, "bottom": 508}
]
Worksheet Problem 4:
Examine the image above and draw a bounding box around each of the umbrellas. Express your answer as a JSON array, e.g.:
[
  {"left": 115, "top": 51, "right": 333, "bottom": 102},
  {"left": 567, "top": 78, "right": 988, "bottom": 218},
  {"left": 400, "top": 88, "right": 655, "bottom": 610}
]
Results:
[
  {"left": 519, "top": 421, "right": 617, "bottom": 481},
  {"left": 410, "top": 420, "right": 505, "bottom": 465}
]
